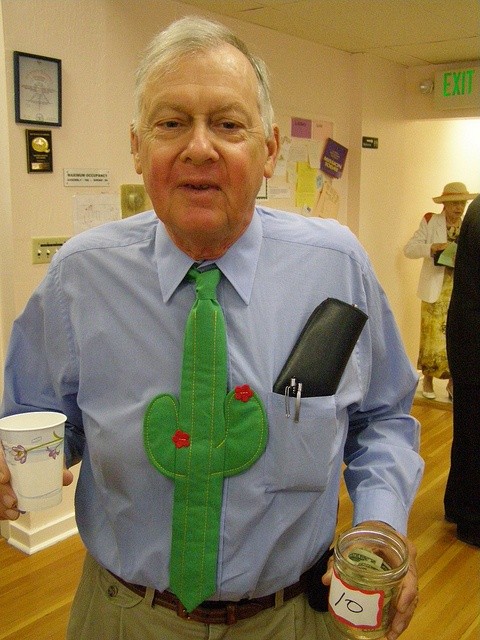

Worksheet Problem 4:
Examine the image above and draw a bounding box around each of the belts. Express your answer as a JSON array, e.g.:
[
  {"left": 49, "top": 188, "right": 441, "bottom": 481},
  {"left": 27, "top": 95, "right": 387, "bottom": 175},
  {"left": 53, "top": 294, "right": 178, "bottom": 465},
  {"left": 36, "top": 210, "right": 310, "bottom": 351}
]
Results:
[{"left": 105, "top": 546, "right": 330, "bottom": 625}]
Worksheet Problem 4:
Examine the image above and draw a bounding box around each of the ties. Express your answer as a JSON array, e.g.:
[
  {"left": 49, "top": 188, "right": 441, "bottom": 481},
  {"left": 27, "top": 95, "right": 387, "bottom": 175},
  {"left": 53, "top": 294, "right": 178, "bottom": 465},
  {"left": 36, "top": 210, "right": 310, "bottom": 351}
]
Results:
[{"left": 144, "top": 269, "right": 267, "bottom": 613}]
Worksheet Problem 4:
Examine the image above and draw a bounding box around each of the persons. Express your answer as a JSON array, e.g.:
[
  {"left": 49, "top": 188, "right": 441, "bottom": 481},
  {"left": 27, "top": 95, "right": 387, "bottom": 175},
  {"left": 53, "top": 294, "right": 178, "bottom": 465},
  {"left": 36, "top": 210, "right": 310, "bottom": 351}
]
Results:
[
  {"left": 405, "top": 183, "right": 480, "bottom": 400},
  {"left": 1, "top": 15, "right": 427, "bottom": 640},
  {"left": 445, "top": 198, "right": 480, "bottom": 548}
]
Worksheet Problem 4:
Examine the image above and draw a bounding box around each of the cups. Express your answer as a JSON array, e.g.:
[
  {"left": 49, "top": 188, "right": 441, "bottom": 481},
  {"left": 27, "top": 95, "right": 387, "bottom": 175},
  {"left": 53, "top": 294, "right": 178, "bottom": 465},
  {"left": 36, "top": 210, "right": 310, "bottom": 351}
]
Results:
[{"left": 1, "top": 408, "right": 67, "bottom": 507}]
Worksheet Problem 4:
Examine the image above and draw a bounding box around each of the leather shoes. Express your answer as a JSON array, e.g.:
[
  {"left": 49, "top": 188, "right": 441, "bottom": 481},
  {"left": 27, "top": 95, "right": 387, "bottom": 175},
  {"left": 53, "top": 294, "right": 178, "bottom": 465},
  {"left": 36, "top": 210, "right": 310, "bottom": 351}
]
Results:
[
  {"left": 445, "top": 505, "right": 464, "bottom": 522},
  {"left": 456, "top": 525, "right": 480, "bottom": 547}
]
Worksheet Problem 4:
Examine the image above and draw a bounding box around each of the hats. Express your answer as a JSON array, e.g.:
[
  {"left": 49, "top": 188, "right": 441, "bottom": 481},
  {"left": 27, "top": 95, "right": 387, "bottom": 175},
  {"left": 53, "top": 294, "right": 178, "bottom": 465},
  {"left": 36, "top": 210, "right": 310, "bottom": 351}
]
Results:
[{"left": 432, "top": 182, "right": 479, "bottom": 203}]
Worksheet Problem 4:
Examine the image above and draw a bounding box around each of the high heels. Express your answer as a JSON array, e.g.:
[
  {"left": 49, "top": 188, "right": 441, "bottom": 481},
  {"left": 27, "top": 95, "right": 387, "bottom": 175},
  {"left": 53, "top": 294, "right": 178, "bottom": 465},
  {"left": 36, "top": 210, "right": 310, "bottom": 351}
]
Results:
[
  {"left": 421, "top": 385, "right": 436, "bottom": 399},
  {"left": 446, "top": 386, "right": 453, "bottom": 399}
]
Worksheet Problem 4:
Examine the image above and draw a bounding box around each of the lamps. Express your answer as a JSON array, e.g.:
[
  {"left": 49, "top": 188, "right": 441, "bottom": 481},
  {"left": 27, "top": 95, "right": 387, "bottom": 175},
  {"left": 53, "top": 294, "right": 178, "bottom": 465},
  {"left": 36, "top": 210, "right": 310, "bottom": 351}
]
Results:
[{"left": 420, "top": 79, "right": 432, "bottom": 93}]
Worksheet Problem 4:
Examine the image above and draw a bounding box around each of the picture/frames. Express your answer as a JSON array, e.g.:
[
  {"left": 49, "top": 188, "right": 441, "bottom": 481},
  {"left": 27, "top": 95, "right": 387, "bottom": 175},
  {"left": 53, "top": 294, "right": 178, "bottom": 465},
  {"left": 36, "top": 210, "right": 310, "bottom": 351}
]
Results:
[{"left": 14, "top": 51, "right": 62, "bottom": 127}]
